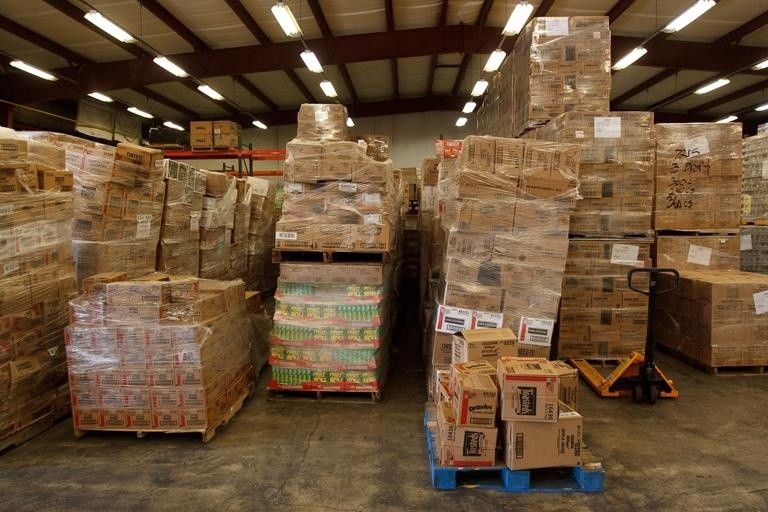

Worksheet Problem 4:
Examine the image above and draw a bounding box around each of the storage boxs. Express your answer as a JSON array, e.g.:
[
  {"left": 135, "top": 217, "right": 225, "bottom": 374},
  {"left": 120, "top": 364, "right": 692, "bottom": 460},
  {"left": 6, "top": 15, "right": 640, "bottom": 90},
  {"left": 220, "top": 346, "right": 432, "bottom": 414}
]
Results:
[
  {"left": 521, "top": 112, "right": 656, "bottom": 164},
  {"left": 476, "top": 15, "right": 613, "bottom": 128},
  {"left": 1, "top": 123, "right": 768, "bottom": 470}
]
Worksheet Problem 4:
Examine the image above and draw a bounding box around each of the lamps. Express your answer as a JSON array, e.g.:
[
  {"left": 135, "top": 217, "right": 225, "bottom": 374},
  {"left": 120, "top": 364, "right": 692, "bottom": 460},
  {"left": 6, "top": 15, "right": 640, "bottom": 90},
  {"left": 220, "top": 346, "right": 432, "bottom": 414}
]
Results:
[
  {"left": 252, "top": 120, "right": 268, "bottom": 130},
  {"left": 300, "top": 49, "right": 325, "bottom": 74},
  {"left": 613, "top": 44, "right": 648, "bottom": 73},
  {"left": 503, "top": 2, "right": 535, "bottom": 38},
  {"left": 267, "top": 2, "right": 305, "bottom": 39},
  {"left": 87, "top": 91, "right": 113, "bottom": 104},
  {"left": 151, "top": 54, "right": 189, "bottom": 79},
  {"left": 82, "top": 8, "right": 137, "bottom": 45},
  {"left": 754, "top": 103, "right": 766, "bottom": 113},
  {"left": 346, "top": 117, "right": 356, "bottom": 128},
  {"left": 197, "top": 84, "right": 225, "bottom": 101},
  {"left": 320, "top": 80, "right": 339, "bottom": 98},
  {"left": 163, "top": 121, "right": 184, "bottom": 130},
  {"left": 471, "top": 80, "right": 489, "bottom": 97},
  {"left": 462, "top": 101, "right": 477, "bottom": 114},
  {"left": 455, "top": 117, "right": 468, "bottom": 128},
  {"left": 9, "top": 59, "right": 58, "bottom": 84},
  {"left": 716, "top": 114, "right": 740, "bottom": 122},
  {"left": 750, "top": 60, "right": 768, "bottom": 74},
  {"left": 694, "top": 76, "right": 733, "bottom": 96},
  {"left": 658, "top": 0, "right": 717, "bottom": 35},
  {"left": 127, "top": 106, "right": 154, "bottom": 118}
]
